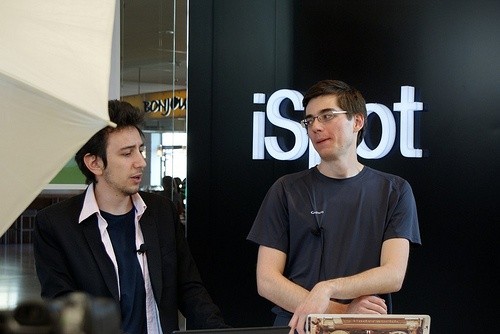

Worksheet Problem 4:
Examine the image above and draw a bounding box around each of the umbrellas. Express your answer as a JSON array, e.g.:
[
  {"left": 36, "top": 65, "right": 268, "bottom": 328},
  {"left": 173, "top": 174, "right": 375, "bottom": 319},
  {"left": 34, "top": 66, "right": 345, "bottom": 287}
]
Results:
[{"left": 0, "top": 0, "right": 117, "bottom": 239}]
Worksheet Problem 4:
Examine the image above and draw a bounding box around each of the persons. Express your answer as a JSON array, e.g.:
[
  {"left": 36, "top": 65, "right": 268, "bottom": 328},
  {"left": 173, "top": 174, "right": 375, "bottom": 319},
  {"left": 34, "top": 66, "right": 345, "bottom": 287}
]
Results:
[
  {"left": 33, "top": 100, "right": 232, "bottom": 334},
  {"left": 153, "top": 176, "right": 184, "bottom": 213},
  {"left": 245, "top": 80, "right": 421, "bottom": 334}
]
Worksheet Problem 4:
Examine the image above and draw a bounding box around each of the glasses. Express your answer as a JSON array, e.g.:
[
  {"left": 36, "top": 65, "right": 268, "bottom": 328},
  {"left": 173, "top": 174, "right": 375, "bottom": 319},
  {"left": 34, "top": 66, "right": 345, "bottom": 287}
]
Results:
[{"left": 303, "top": 110, "right": 347, "bottom": 127}]
